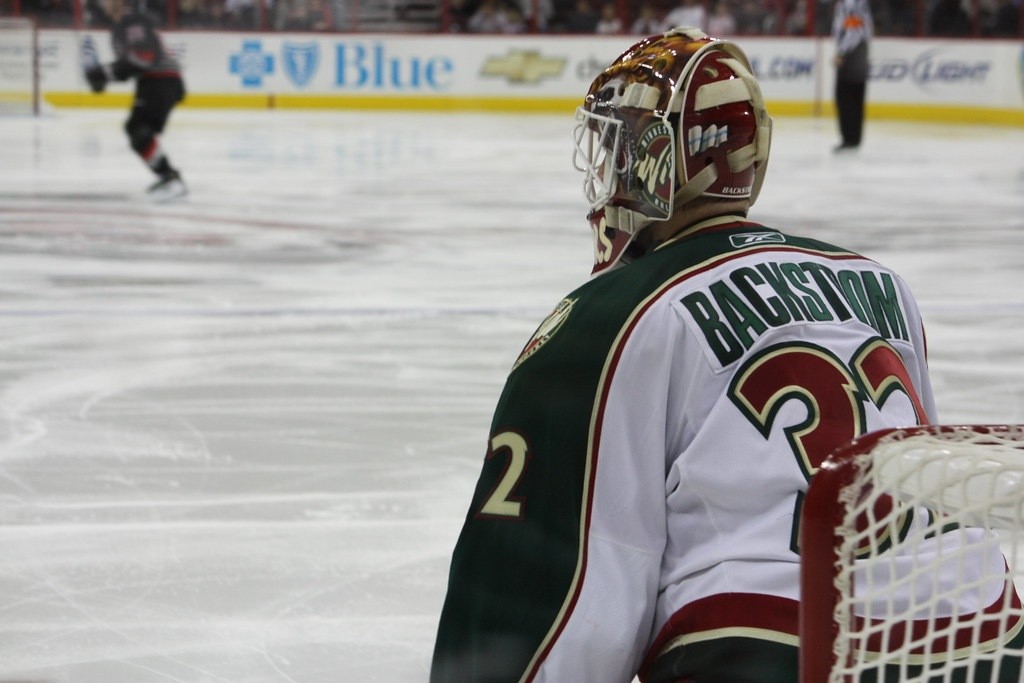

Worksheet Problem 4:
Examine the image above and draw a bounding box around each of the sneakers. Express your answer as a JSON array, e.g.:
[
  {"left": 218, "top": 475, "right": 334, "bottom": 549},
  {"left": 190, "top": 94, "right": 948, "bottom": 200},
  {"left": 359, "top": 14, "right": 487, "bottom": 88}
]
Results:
[{"left": 147, "top": 157, "right": 184, "bottom": 199}]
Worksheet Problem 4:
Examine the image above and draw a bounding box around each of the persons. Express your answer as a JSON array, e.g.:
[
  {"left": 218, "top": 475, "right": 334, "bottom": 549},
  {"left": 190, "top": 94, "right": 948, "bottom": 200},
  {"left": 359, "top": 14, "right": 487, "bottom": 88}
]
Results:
[
  {"left": 431, "top": 24, "right": 1024, "bottom": 683},
  {"left": 832, "top": 0, "right": 871, "bottom": 148},
  {"left": 0, "top": 0, "right": 1024, "bottom": 38},
  {"left": 87, "top": 0, "right": 185, "bottom": 193}
]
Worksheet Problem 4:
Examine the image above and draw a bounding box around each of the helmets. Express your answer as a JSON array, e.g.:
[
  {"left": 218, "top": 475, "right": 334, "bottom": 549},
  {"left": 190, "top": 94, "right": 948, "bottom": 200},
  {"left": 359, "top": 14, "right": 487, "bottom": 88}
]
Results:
[{"left": 571, "top": 25, "right": 773, "bottom": 280}]
